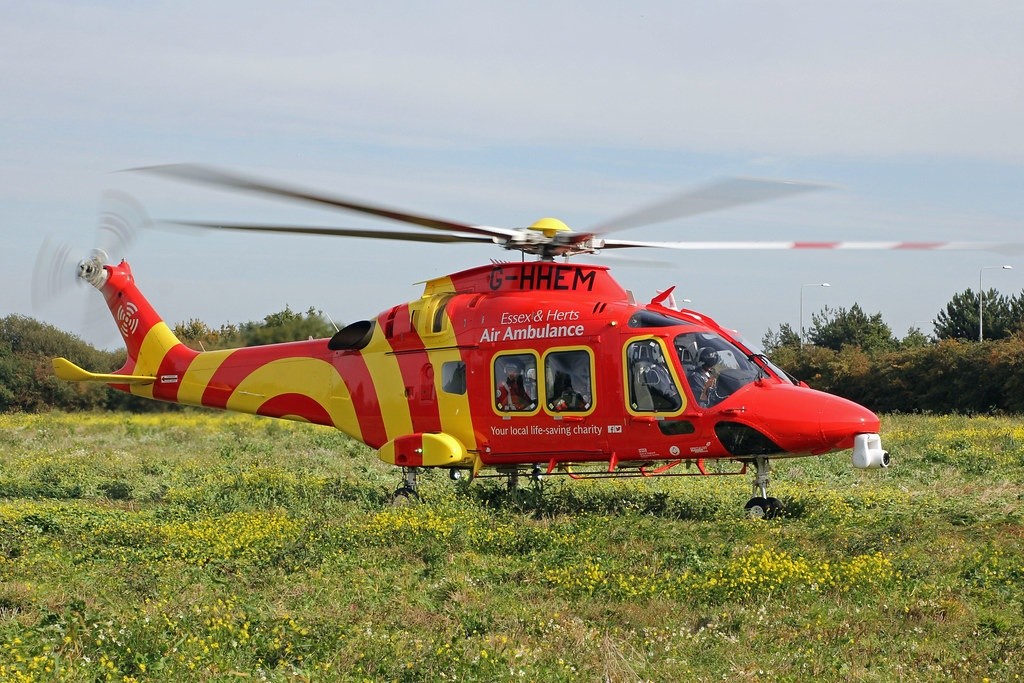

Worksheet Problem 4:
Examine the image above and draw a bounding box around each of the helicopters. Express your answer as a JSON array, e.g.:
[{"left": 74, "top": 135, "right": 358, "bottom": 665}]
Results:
[{"left": 53, "top": 166, "right": 1015, "bottom": 520}]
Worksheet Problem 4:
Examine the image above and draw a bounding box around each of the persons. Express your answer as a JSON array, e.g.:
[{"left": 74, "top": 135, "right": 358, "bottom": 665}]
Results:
[
  {"left": 692, "top": 346, "right": 719, "bottom": 402},
  {"left": 496, "top": 360, "right": 532, "bottom": 411},
  {"left": 648, "top": 364, "right": 682, "bottom": 410}
]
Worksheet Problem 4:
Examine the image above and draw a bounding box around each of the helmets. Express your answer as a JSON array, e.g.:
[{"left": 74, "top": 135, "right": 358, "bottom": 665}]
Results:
[
  {"left": 652, "top": 344, "right": 665, "bottom": 363},
  {"left": 694, "top": 347, "right": 718, "bottom": 367},
  {"left": 504, "top": 362, "right": 518, "bottom": 374}
]
